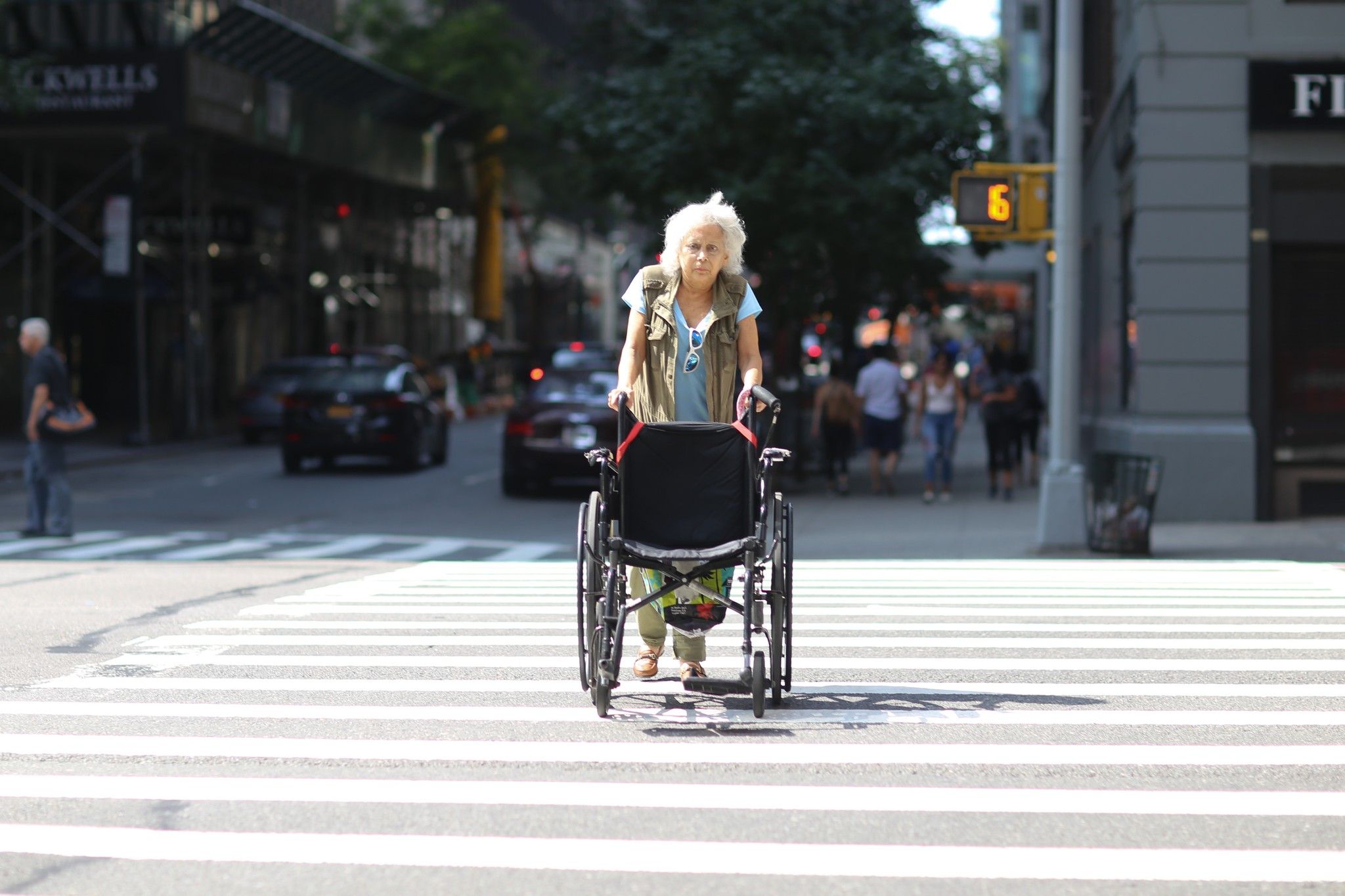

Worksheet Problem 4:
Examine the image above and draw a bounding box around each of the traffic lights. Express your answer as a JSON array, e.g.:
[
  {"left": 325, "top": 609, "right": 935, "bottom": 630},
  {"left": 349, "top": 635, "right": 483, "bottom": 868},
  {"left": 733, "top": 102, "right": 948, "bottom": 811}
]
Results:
[{"left": 949, "top": 167, "right": 1016, "bottom": 236}]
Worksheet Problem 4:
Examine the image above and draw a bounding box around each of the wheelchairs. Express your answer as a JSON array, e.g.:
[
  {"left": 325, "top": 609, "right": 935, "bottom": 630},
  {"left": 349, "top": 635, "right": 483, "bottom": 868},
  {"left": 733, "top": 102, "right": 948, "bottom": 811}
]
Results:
[{"left": 576, "top": 384, "right": 795, "bottom": 719}]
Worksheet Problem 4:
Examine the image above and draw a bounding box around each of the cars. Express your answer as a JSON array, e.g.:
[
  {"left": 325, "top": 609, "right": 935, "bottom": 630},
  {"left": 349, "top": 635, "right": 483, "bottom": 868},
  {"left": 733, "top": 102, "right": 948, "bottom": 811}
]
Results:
[
  {"left": 500, "top": 365, "right": 635, "bottom": 499},
  {"left": 236, "top": 356, "right": 378, "bottom": 445},
  {"left": 280, "top": 348, "right": 448, "bottom": 475}
]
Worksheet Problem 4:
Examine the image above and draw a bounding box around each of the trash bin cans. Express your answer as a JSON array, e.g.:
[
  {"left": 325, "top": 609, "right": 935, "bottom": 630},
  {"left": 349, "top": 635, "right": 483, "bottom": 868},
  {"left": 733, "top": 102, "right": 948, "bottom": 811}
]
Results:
[{"left": 1087, "top": 452, "right": 1166, "bottom": 554}]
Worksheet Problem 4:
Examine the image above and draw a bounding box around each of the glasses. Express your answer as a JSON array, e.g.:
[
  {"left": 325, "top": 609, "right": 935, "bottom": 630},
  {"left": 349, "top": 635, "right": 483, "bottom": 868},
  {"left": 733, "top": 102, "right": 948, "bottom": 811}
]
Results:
[{"left": 683, "top": 327, "right": 703, "bottom": 374}]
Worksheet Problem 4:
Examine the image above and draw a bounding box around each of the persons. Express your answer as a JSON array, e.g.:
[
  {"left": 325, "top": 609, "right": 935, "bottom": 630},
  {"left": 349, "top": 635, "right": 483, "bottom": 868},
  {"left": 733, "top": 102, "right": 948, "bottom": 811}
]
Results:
[
  {"left": 809, "top": 341, "right": 1045, "bottom": 501},
  {"left": 14, "top": 319, "right": 80, "bottom": 536},
  {"left": 609, "top": 191, "right": 764, "bottom": 679}
]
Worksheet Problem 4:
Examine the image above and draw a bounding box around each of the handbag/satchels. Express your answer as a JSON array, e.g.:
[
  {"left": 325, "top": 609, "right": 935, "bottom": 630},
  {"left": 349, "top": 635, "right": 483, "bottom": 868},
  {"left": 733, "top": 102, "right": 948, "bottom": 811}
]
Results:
[{"left": 43, "top": 402, "right": 96, "bottom": 435}]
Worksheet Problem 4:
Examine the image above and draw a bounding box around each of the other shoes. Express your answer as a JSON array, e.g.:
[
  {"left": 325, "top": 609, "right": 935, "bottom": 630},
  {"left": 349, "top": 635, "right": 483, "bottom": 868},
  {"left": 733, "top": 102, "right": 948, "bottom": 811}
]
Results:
[
  {"left": 679, "top": 662, "right": 706, "bottom": 681},
  {"left": 923, "top": 491, "right": 935, "bottom": 501},
  {"left": 990, "top": 484, "right": 998, "bottom": 495},
  {"left": 634, "top": 645, "right": 665, "bottom": 678},
  {"left": 1005, "top": 488, "right": 1011, "bottom": 500},
  {"left": 938, "top": 493, "right": 950, "bottom": 501},
  {"left": 20, "top": 527, "right": 45, "bottom": 536}
]
